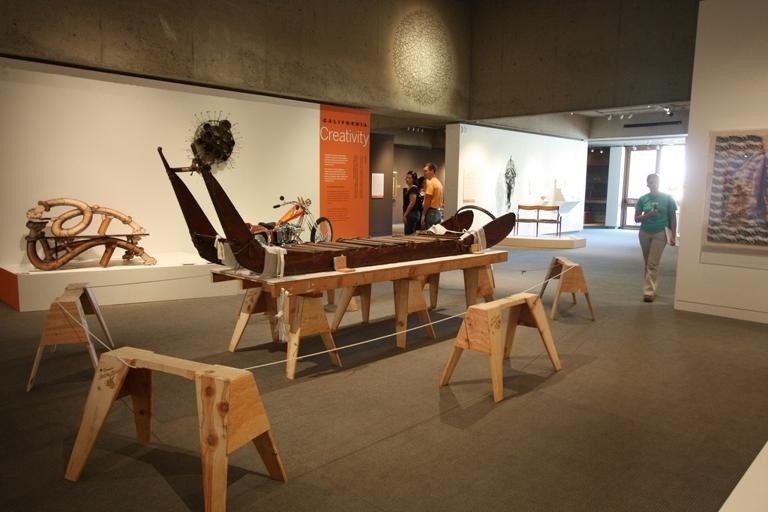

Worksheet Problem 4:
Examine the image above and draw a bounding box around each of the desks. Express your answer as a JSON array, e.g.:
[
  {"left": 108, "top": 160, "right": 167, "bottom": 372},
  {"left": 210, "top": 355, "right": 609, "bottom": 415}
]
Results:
[{"left": 212, "top": 247, "right": 507, "bottom": 382}]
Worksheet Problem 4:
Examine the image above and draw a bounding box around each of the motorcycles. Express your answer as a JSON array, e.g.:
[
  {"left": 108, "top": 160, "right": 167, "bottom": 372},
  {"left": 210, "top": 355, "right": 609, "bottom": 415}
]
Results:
[{"left": 244, "top": 195, "right": 334, "bottom": 247}]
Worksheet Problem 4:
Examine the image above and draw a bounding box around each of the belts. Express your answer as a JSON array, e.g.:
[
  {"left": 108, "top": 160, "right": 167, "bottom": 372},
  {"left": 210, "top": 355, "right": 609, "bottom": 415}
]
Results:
[{"left": 430, "top": 207, "right": 440, "bottom": 210}]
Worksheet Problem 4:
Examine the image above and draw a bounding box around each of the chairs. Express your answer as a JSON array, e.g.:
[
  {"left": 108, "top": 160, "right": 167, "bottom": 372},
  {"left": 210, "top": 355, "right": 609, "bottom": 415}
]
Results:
[{"left": 513, "top": 205, "right": 562, "bottom": 236}]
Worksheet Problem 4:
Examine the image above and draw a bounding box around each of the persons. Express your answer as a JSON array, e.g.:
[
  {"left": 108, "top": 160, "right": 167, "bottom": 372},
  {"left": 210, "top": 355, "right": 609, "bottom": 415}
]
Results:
[
  {"left": 633, "top": 172, "right": 680, "bottom": 303},
  {"left": 419, "top": 162, "right": 445, "bottom": 229},
  {"left": 416, "top": 175, "right": 426, "bottom": 211},
  {"left": 401, "top": 169, "right": 423, "bottom": 235}
]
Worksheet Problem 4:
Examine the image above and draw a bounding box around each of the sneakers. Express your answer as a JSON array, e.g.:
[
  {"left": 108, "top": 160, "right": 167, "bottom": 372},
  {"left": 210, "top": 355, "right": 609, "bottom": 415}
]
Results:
[{"left": 644, "top": 291, "right": 656, "bottom": 302}]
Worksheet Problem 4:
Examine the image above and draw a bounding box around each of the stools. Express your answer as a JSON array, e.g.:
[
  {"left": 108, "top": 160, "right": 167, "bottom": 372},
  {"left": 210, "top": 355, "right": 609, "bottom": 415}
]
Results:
[
  {"left": 65, "top": 346, "right": 287, "bottom": 512},
  {"left": 540, "top": 257, "right": 597, "bottom": 322},
  {"left": 440, "top": 292, "right": 561, "bottom": 403},
  {"left": 26, "top": 282, "right": 114, "bottom": 392}
]
